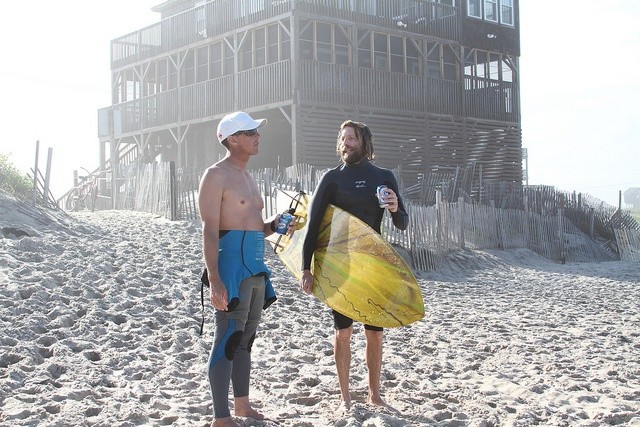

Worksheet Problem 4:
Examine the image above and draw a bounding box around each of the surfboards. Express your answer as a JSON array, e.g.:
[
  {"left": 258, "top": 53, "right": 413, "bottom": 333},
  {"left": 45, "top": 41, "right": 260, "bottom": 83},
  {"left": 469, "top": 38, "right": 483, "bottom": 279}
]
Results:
[{"left": 265, "top": 189, "right": 425, "bottom": 328}]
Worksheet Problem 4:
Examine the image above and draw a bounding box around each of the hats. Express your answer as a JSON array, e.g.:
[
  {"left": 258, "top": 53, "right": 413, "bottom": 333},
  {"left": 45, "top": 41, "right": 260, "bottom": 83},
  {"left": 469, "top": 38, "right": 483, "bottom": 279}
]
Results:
[{"left": 217, "top": 111, "right": 267, "bottom": 144}]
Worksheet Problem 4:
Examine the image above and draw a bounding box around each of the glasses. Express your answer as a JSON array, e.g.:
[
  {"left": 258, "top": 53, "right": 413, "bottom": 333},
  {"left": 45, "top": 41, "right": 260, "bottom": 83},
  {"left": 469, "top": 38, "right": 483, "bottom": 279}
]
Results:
[{"left": 238, "top": 129, "right": 257, "bottom": 136}]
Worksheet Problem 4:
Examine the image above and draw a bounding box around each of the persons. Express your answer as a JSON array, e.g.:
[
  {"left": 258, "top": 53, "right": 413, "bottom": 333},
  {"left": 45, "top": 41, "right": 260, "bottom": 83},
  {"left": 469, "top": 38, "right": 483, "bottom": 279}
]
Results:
[
  {"left": 299, "top": 119, "right": 409, "bottom": 413},
  {"left": 197, "top": 110, "right": 297, "bottom": 427}
]
